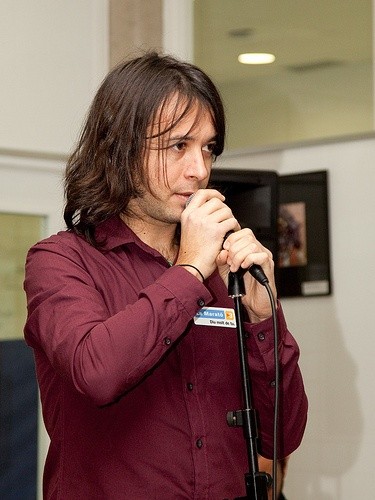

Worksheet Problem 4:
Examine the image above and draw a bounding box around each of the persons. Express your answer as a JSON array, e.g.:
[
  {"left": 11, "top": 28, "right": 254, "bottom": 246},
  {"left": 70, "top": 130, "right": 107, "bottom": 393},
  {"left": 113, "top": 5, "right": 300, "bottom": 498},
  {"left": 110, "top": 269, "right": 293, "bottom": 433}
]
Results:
[
  {"left": 20, "top": 50, "right": 307, "bottom": 500},
  {"left": 256, "top": 452, "right": 287, "bottom": 499}
]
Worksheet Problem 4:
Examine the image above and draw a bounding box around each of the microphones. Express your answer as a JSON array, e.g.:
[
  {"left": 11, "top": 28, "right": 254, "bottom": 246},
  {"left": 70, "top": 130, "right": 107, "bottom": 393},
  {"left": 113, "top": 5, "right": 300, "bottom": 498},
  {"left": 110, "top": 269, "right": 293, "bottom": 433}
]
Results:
[{"left": 184, "top": 192, "right": 268, "bottom": 287}]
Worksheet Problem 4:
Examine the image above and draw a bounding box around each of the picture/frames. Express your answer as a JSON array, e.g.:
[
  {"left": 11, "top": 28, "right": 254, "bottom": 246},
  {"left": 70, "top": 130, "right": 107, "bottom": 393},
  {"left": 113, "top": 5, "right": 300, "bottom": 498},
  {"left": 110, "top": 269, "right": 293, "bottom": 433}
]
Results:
[{"left": 276, "top": 200, "right": 309, "bottom": 269}]
